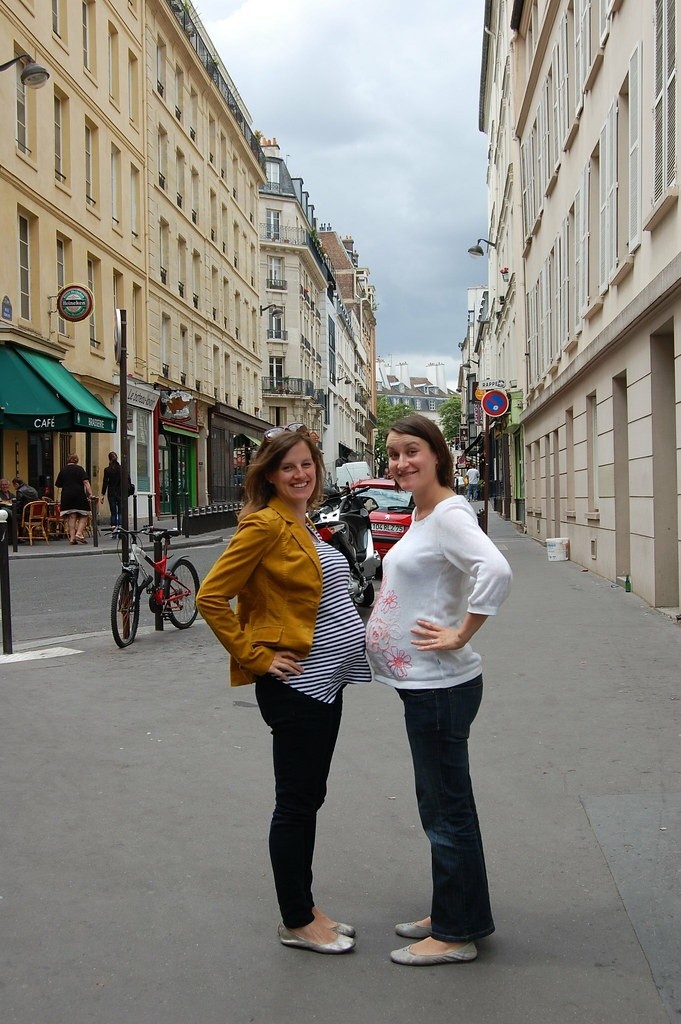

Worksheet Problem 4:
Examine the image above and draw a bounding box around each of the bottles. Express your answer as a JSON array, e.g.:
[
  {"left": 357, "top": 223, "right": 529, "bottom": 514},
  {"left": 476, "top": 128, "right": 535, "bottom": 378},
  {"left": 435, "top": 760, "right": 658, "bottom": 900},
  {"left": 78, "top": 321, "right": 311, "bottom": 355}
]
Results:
[{"left": 625, "top": 575, "right": 630, "bottom": 592}]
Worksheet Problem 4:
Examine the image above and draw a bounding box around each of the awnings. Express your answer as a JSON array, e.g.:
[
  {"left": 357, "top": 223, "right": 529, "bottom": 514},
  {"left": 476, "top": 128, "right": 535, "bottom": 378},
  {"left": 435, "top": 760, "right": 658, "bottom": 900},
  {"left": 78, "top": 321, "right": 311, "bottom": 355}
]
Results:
[
  {"left": 0, "top": 344, "right": 118, "bottom": 433},
  {"left": 243, "top": 433, "right": 261, "bottom": 446}
]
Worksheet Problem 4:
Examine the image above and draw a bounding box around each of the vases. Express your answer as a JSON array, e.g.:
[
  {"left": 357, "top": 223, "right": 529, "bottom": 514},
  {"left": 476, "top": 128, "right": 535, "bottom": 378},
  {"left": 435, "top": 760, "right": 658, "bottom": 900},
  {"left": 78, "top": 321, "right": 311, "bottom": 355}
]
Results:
[{"left": 502, "top": 273, "right": 509, "bottom": 282}]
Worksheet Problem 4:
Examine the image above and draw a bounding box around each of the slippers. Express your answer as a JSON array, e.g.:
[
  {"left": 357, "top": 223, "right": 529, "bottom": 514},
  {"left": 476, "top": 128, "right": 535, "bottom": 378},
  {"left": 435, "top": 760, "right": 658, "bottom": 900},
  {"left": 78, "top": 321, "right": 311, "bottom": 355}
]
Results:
[
  {"left": 70, "top": 538, "right": 78, "bottom": 544},
  {"left": 74, "top": 534, "right": 88, "bottom": 544}
]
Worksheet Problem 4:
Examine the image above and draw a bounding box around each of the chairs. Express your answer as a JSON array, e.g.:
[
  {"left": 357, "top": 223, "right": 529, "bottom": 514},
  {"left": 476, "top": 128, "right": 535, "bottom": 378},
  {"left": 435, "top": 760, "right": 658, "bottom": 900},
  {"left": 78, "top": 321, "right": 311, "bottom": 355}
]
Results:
[{"left": 16, "top": 496, "right": 101, "bottom": 547}]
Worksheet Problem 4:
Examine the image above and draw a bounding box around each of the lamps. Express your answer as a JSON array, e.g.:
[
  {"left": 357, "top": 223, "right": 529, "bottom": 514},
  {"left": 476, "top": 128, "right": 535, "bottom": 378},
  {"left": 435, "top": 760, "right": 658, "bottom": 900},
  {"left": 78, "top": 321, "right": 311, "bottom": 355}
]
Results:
[
  {"left": 0, "top": 54, "right": 50, "bottom": 90},
  {"left": 467, "top": 239, "right": 495, "bottom": 258},
  {"left": 337, "top": 376, "right": 352, "bottom": 385},
  {"left": 462, "top": 359, "right": 480, "bottom": 371},
  {"left": 260, "top": 303, "right": 284, "bottom": 319}
]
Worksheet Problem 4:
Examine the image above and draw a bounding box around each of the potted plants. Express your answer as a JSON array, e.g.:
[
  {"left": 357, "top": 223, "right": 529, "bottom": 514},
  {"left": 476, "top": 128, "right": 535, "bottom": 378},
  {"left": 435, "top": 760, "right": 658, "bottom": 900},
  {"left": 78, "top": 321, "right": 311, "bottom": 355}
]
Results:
[{"left": 283, "top": 375, "right": 290, "bottom": 394}]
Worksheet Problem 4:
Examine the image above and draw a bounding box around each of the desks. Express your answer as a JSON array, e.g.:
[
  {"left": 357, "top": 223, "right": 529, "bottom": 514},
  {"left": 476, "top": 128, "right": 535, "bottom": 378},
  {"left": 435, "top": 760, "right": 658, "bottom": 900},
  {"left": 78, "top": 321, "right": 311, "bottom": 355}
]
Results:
[{"left": 0, "top": 501, "right": 61, "bottom": 517}]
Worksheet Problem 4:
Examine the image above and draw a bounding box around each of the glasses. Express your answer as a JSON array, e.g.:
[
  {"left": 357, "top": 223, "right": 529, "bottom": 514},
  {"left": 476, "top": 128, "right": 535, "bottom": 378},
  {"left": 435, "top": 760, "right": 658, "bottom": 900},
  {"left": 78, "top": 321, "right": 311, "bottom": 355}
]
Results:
[{"left": 258, "top": 422, "right": 309, "bottom": 460}]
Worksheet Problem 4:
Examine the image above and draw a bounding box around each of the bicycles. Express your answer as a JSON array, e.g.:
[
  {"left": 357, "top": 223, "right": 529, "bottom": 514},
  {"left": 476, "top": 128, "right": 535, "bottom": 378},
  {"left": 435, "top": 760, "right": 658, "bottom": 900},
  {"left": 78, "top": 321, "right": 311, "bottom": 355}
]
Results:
[{"left": 101, "top": 524, "right": 200, "bottom": 649}]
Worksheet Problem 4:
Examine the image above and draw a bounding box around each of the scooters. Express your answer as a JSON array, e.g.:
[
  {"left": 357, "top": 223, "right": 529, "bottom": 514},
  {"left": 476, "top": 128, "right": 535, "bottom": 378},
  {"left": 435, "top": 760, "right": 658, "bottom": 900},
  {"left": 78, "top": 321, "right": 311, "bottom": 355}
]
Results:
[{"left": 306, "top": 481, "right": 381, "bottom": 607}]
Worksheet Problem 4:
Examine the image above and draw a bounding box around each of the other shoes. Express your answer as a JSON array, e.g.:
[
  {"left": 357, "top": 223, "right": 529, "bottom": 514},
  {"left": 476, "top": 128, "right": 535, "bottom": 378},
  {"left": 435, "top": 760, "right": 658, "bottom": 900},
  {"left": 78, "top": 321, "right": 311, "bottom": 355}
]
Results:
[
  {"left": 277, "top": 920, "right": 357, "bottom": 952},
  {"left": 390, "top": 937, "right": 478, "bottom": 965},
  {"left": 328, "top": 921, "right": 355, "bottom": 937},
  {"left": 395, "top": 920, "right": 432, "bottom": 938}
]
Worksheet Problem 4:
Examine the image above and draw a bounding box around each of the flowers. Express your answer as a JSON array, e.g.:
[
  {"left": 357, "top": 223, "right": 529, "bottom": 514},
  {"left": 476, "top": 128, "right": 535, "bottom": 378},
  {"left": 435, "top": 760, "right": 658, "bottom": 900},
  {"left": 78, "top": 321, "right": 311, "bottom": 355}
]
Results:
[{"left": 500, "top": 267, "right": 508, "bottom": 274}]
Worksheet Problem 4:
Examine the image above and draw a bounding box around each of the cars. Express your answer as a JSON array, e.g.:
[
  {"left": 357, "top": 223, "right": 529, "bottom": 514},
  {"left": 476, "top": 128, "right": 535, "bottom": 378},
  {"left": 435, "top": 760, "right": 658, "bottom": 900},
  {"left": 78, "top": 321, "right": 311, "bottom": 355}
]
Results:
[{"left": 349, "top": 478, "right": 416, "bottom": 563}]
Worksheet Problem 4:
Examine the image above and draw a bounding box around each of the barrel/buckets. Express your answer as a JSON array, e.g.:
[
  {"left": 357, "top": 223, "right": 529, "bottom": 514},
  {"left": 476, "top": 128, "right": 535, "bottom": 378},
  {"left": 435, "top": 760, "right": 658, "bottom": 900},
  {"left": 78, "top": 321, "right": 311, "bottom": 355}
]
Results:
[{"left": 546, "top": 537, "right": 569, "bottom": 562}]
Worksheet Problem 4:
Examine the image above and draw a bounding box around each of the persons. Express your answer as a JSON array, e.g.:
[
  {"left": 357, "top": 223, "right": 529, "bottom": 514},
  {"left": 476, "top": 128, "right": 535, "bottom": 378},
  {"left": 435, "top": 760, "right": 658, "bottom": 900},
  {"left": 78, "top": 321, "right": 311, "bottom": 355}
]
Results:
[
  {"left": 196, "top": 423, "right": 372, "bottom": 953},
  {"left": 365, "top": 413, "right": 512, "bottom": 964},
  {"left": 0, "top": 478, "right": 15, "bottom": 501},
  {"left": 55, "top": 455, "right": 93, "bottom": 545},
  {"left": 12, "top": 477, "right": 38, "bottom": 522},
  {"left": 465, "top": 463, "right": 480, "bottom": 502},
  {"left": 100, "top": 452, "right": 131, "bottom": 539}
]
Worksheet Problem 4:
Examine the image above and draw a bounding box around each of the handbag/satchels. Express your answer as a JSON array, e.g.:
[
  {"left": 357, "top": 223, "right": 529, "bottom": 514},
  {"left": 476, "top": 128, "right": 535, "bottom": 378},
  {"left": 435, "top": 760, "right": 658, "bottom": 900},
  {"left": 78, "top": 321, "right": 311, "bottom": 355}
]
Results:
[{"left": 128, "top": 483, "right": 135, "bottom": 497}]
